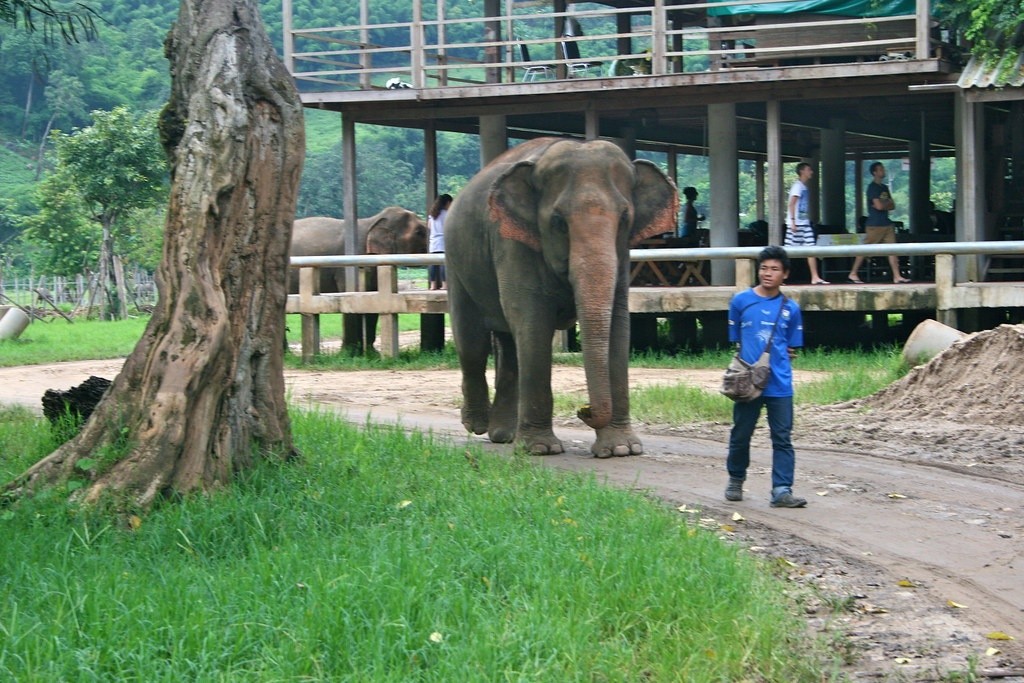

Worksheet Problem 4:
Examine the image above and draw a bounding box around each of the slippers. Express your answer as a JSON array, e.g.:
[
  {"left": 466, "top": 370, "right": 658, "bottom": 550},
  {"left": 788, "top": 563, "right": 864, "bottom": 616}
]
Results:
[
  {"left": 846, "top": 275, "right": 867, "bottom": 285},
  {"left": 894, "top": 277, "right": 914, "bottom": 284},
  {"left": 811, "top": 277, "right": 830, "bottom": 285}
]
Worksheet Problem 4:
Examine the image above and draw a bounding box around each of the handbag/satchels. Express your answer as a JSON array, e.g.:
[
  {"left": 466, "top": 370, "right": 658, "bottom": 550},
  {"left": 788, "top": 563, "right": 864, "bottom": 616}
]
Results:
[{"left": 719, "top": 351, "right": 771, "bottom": 404}]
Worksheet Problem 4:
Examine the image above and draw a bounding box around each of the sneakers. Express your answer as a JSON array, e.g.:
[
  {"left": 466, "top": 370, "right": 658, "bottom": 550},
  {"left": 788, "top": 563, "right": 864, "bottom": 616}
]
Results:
[
  {"left": 770, "top": 493, "right": 806, "bottom": 508},
  {"left": 725, "top": 478, "right": 743, "bottom": 501}
]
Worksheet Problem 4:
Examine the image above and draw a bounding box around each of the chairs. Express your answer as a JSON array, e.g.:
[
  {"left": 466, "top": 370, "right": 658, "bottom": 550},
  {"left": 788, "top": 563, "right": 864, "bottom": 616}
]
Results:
[
  {"left": 516, "top": 36, "right": 556, "bottom": 82},
  {"left": 560, "top": 34, "right": 603, "bottom": 80}
]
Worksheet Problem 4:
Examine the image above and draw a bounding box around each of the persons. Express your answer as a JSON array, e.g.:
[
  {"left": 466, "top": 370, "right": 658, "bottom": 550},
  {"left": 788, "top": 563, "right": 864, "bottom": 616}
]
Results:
[
  {"left": 929, "top": 202, "right": 947, "bottom": 233},
  {"left": 681, "top": 187, "right": 705, "bottom": 236},
  {"left": 726, "top": 246, "right": 808, "bottom": 507},
  {"left": 783, "top": 162, "right": 830, "bottom": 285},
  {"left": 428, "top": 194, "right": 453, "bottom": 290},
  {"left": 848, "top": 162, "right": 911, "bottom": 284}
]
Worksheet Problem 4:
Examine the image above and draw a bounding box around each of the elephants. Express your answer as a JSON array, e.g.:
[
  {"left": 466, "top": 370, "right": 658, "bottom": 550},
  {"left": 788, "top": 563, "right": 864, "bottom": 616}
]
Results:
[
  {"left": 448, "top": 139, "right": 680, "bottom": 458},
  {"left": 284, "top": 204, "right": 429, "bottom": 355}
]
